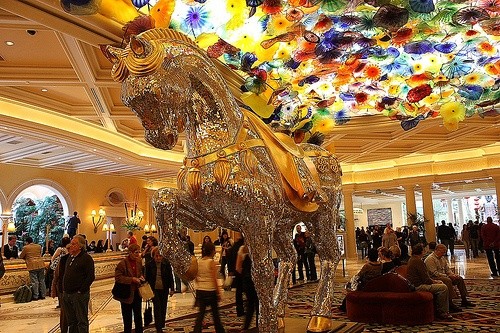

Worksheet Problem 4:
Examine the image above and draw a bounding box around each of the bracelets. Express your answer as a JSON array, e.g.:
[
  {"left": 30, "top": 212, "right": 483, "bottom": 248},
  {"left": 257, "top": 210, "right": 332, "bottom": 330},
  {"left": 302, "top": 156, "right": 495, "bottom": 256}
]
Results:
[{"left": 302, "top": 241, "right": 304, "bottom": 243}]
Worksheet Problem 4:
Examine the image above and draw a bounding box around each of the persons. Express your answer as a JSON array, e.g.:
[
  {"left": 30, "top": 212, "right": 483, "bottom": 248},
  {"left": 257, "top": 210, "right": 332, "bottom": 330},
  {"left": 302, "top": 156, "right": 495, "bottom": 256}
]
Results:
[
  {"left": 49, "top": 233, "right": 95, "bottom": 333},
  {"left": 0, "top": 236, "right": 18, "bottom": 260},
  {"left": 296, "top": 231, "right": 318, "bottom": 282},
  {"left": 422, "top": 220, "right": 459, "bottom": 262},
  {"left": 146, "top": 246, "right": 175, "bottom": 333},
  {"left": 424, "top": 244, "right": 476, "bottom": 313},
  {"left": 461, "top": 219, "right": 500, "bottom": 258},
  {"left": 271, "top": 241, "right": 299, "bottom": 286},
  {"left": 172, "top": 233, "right": 194, "bottom": 292},
  {"left": 18, "top": 237, "right": 46, "bottom": 299},
  {"left": 228, "top": 238, "right": 260, "bottom": 333},
  {"left": 79, "top": 234, "right": 114, "bottom": 253},
  {"left": 117, "top": 230, "right": 158, "bottom": 301},
  {"left": 356, "top": 223, "right": 420, "bottom": 274},
  {"left": 200, "top": 229, "right": 228, "bottom": 255},
  {"left": 292, "top": 225, "right": 310, "bottom": 280},
  {"left": 219, "top": 232, "right": 234, "bottom": 286},
  {"left": 481, "top": 217, "right": 500, "bottom": 276},
  {"left": 115, "top": 244, "right": 145, "bottom": 333},
  {"left": 194, "top": 242, "right": 226, "bottom": 333},
  {"left": 65, "top": 212, "right": 81, "bottom": 240},
  {"left": 337, "top": 248, "right": 383, "bottom": 312},
  {"left": 42, "top": 239, "right": 55, "bottom": 257},
  {"left": 406, "top": 243, "right": 453, "bottom": 320}
]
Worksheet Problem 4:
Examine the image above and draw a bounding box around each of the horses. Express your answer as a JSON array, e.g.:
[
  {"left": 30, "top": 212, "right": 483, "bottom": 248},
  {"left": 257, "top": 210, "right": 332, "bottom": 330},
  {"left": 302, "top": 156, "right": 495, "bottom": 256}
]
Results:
[{"left": 106, "top": 27, "right": 343, "bottom": 333}]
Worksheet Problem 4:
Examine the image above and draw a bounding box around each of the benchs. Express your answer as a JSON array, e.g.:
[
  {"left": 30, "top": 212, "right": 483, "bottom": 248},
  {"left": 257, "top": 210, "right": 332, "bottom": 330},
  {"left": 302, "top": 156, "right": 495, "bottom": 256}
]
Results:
[{"left": 344, "top": 260, "right": 437, "bottom": 325}]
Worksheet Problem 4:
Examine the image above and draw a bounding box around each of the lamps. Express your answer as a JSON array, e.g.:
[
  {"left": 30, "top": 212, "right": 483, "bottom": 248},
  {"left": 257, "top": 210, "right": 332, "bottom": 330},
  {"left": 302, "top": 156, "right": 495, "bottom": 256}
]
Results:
[
  {"left": 143, "top": 223, "right": 158, "bottom": 235},
  {"left": 90, "top": 208, "right": 116, "bottom": 251}
]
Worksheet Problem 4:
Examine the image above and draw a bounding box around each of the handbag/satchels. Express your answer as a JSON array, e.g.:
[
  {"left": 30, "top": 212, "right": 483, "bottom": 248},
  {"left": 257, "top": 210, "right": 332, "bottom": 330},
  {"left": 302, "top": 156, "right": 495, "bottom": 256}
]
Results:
[
  {"left": 139, "top": 278, "right": 155, "bottom": 302},
  {"left": 111, "top": 260, "right": 131, "bottom": 301},
  {"left": 143, "top": 299, "right": 153, "bottom": 328}
]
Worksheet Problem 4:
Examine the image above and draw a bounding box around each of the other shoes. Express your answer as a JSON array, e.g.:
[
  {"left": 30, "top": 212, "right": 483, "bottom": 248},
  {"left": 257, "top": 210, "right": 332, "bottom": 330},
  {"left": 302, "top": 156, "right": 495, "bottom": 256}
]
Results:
[
  {"left": 449, "top": 303, "right": 462, "bottom": 312},
  {"left": 241, "top": 320, "right": 251, "bottom": 331},
  {"left": 297, "top": 278, "right": 303, "bottom": 281},
  {"left": 40, "top": 296, "right": 45, "bottom": 299},
  {"left": 174, "top": 290, "right": 182, "bottom": 293},
  {"left": 435, "top": 311, "right": 453, "bottom": 320},
  {"left": 460, "top": 300, "right": 476, "bottom": 307},
  {"left": 491, "top": 273, "right": 499, "bottom": 277},
  {"left": 337, "top": 306, "right": 346, "bottom": 312},
  {"left": 57, "top": 305, "right": 61, "bottom": 308}
]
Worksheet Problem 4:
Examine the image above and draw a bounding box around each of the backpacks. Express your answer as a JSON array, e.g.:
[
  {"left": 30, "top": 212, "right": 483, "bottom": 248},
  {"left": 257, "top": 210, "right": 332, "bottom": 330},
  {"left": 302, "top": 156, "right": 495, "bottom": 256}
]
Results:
[{"left": 14, "top": 285, "right": 32, "bottom": 304}]
